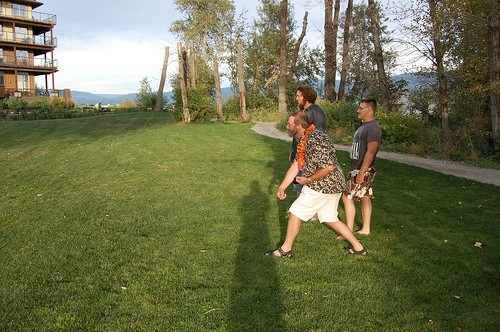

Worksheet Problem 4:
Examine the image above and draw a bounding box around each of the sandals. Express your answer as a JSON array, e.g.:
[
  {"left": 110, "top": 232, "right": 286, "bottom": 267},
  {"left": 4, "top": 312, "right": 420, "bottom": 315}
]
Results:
[
  {"left": 343, "top": 248, "right": 367, "bottom": 256},
  {"left": 265, "top": 248, "right": 292, "bottom": 258}
]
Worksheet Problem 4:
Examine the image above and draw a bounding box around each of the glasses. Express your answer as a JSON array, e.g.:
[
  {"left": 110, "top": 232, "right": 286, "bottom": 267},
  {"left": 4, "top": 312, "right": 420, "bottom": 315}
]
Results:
[{"left": 358, "top": 106, "right": 368, "bottom": 110}]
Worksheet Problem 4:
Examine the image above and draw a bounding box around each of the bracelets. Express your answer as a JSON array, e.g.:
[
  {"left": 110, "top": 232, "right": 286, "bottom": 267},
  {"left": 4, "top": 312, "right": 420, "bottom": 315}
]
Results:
[{"left": 308, "top": 177, "right": 312, "bottom": 184}]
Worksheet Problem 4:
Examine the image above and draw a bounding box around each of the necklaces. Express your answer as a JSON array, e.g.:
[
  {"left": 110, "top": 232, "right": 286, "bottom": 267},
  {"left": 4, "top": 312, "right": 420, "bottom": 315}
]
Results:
[{"left": 296, "top": 123, "right": 316, "bottom": 171}]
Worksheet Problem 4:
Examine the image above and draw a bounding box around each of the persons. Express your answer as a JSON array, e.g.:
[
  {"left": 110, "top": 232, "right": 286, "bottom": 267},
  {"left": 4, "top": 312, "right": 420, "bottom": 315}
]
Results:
[
  {"left": 264, "top": 113, "right": 368, "bottom": 259},
  {"left": 336, "top": 99, "right": 383, "bottom": 240},
  {"left": 288, "top": 85, "right": 328, "bottom": 223}
]
